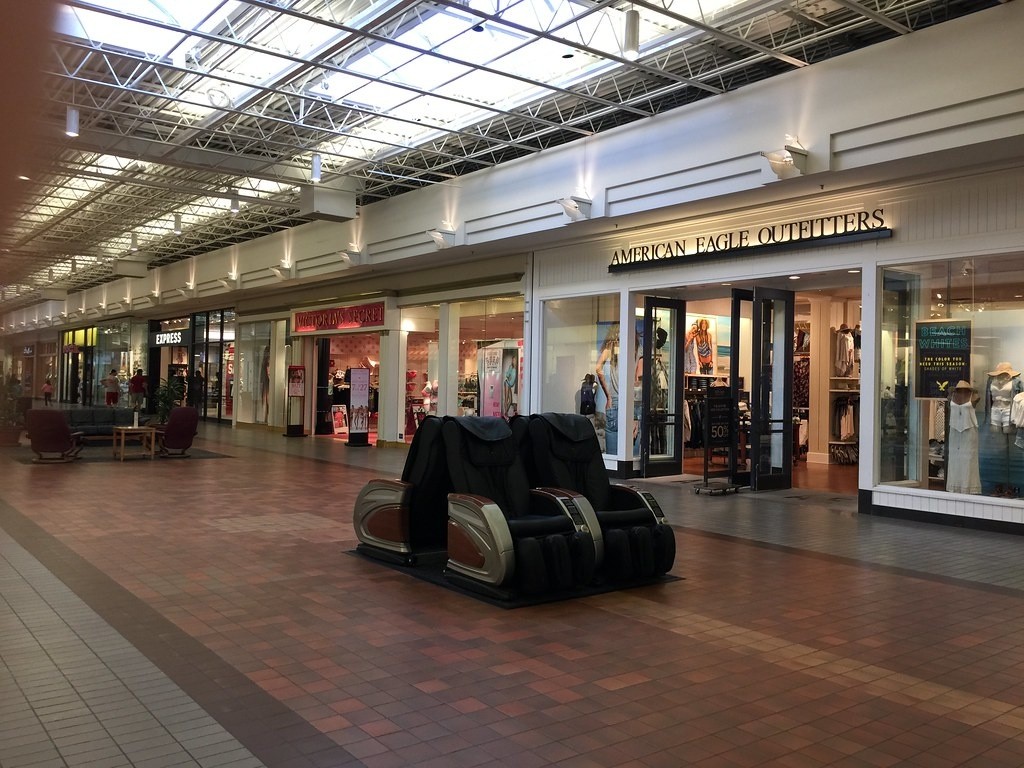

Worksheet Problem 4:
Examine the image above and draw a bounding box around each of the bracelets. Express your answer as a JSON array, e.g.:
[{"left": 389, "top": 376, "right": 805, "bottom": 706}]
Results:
[{"left": 710, "top": 361, "right": 713, "bottom": 363}]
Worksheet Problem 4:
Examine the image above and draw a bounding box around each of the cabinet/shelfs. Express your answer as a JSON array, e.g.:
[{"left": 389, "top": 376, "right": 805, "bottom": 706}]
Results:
[{"left": 829, "top": 377, "right": 860, "bottom": 444}]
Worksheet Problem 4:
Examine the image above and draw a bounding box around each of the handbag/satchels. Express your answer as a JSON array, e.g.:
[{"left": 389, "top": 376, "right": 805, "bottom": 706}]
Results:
[{"left": 100, "top": 377, "right": 116, "bottom": 389}]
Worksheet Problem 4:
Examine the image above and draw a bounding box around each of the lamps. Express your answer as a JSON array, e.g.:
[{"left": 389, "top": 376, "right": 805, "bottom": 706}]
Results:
[
  {"left": 174, "top": 213, "right": 182, "bottom": 234},
  {"left": 29, "top": 277, "right": 35, "bottom": 290},
  {"left": 97, "top": 248, "right": 103, "bottom": 264},
  {"left": 66, "top": 81, "right": 79, "bottom": 137},
  {"left": 232, "top": 177, "right": 239, "bottom": 211},
  {"left": 132, "top": 234, "right": 138, "bottom": 252},
  {"left": 16, "top": 285, "right": 21, "bottom": 295},
  {"left": 1, "top": 291, "right": 5, "bottom": 301},
  {"left": 72, "top": 259, "right": 78, "bottom": 274},
  {"left": 624, "top": 1, "right": 640, "bottom": 60},
  {"left": 311, "top": 151, "right": 321, "bottom": 183},
  {"left": 48, "top": 269, "right": 54, "bottom": 282}
]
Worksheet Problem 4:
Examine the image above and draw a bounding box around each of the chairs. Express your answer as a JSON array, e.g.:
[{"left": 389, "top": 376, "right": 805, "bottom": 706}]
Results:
[
  {"left": 26, "top": 409, "right": 84, "bottom": 463},
  {"left": 352, "top": 410, "right": 678, "bottom": 605},
  {"left": 152, "top": 407, "right": 199, "bottom": 458}
]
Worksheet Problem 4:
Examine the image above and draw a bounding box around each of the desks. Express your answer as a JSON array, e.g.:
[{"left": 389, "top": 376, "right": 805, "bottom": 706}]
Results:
[{"left": 113, "top": 427, "right": 157, "bottom": 461}]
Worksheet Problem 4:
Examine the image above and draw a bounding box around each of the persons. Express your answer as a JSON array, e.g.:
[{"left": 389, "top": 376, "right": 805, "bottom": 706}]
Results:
[
  {"left": 259, "top": 346, "right": 270, "bottom": 422},
  {"left": 835, "top": 324, "right": 861, "bottom": 376},
  {"left": 350, "top": 404, "right": 368, "bottom": 430},
  {"left": 683, "top": 318, "right": 713, "bottom": 374},
  {"left": 580, "top": 374, "right": 598, "bottom": 420},
  {"left": 945, "top": 380, "right": 982, "bottom": 495},
  {"left": 984, "top": 363, "right": 1024, "bottom": 434},
  {"left": 189, "top": 370, "right": 204, "bottom": 416},
  {"left": 503, "top": 356, "right": 516, "bottom": 417},
  {"left": 129, "top": 369, "right": 145, "bottom": 411},
  {"left": 100, "top": 370, "right": 120, "bottom": 408},
  {"left": 334, "top": 407, "right": 343, "bottom": 427},
  {"left": 1011, "top": 391, "right": 1024, "bottom": 449},
  {"left": 290, "top": 370, "right": 303, "bottom": 383},
  {"left": 41, "top": 380, "right": 53, "bottom": 406},
  {"left": 596, "top": 321, "right": 644, "bottom": 457},
  {"left": 10, "top": 374, "right": 22, "bottom": 392}
]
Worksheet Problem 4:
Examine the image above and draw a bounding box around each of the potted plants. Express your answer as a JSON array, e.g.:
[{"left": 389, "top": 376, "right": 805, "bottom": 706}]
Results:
[
  {"left": 0, "top": 384, "right": 30, "bottom": 446},
  {"left": 147, "top": 378, "right": 186, "bottom": 436}
]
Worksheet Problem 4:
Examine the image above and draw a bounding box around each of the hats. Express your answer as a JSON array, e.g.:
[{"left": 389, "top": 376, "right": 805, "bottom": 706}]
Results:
[
  {"left": 697, "top": 317, "right": 710, "bottom": 330},
  {"left": 952, "top": 379, "right": 974, "bottom": 389},
  {"left": 987, "top": 362, "right": 1022, "bottom": 378},
  {"left": 836, "top": 323, "right": 853, "bottom": 332},
  {"left": 853, "top": 324, "right": 861, "bottom": 331}
]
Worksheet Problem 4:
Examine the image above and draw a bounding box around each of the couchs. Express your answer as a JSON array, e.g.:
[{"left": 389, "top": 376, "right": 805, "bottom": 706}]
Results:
[{"left": 55, "top": 407, "right": 144, "bottom": 443}]
